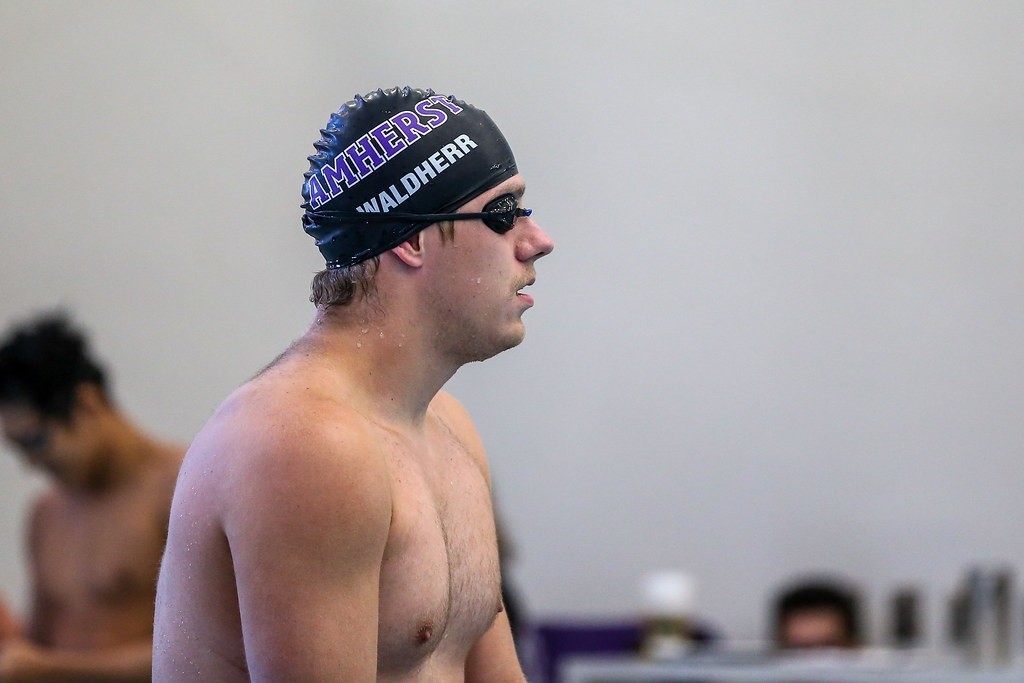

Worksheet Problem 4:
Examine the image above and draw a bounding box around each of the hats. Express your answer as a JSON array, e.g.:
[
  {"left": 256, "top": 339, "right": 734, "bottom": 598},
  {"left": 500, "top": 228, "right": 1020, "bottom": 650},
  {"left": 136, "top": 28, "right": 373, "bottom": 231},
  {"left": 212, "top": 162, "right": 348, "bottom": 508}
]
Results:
[{"left": 300, "top": 86, "right": 520, "bottom": 270}]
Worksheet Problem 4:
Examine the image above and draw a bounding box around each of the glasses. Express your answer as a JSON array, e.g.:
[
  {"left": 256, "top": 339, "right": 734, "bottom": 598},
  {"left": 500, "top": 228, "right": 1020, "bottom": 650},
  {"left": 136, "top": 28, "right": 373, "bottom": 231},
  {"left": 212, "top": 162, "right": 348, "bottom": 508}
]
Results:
[{"left": 308, "top": 194, "right": 533, "bottom": 235}]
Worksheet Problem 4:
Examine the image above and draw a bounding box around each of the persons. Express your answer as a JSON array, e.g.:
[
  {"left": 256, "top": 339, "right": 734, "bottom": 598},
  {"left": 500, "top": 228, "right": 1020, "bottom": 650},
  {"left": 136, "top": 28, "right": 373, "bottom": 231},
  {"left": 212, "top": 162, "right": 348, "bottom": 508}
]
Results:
[
  {"left": 770, "top": 581, "right": 862, "bottom": 653},
  {"left": 0, "top": 310, "right": 192, "bottom": 682},
  {"left": 153, "top": 83, "right": 553, "bottom": 682}
]
